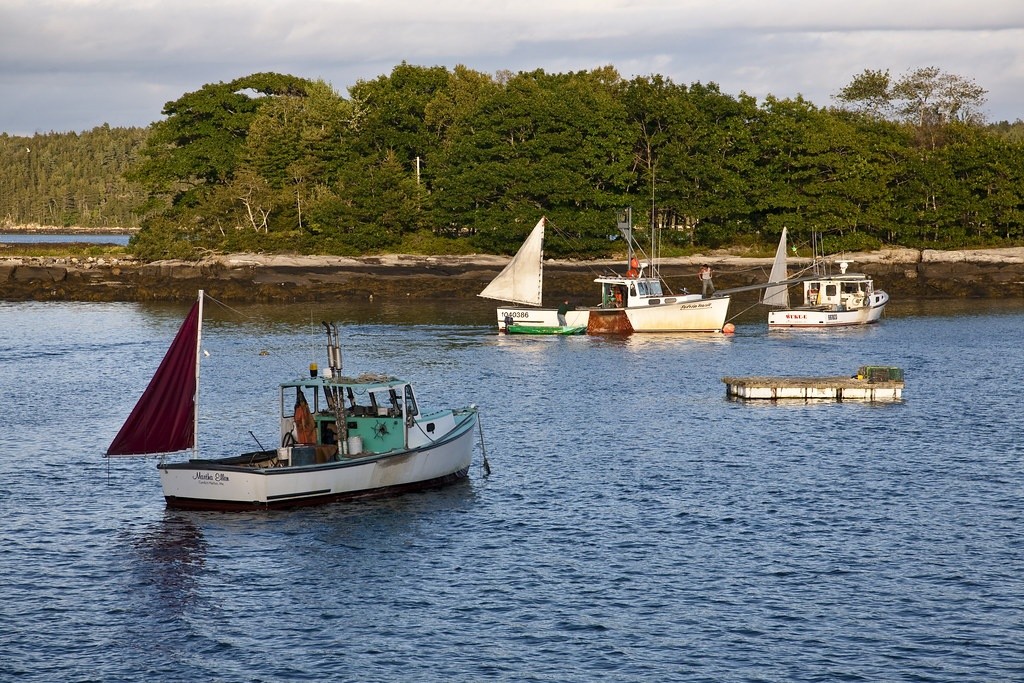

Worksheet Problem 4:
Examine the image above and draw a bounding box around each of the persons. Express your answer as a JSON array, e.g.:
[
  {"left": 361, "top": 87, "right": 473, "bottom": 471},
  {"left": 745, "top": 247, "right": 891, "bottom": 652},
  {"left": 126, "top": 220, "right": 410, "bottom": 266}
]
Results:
[
  {"left": 698, "top": 263, "right": 716, "bottom": 299},
  {"left": 557, "top": 300, "right": 576, "bottom": 327}
]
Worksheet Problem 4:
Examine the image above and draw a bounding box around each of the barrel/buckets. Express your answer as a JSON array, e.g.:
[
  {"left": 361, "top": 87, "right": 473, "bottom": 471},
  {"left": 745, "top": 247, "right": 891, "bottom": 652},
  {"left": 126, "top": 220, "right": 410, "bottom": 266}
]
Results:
[
  {"left": 348, "top": 436, "right": 362, "bottom": 454},
  {"left": 277, "top": 448, "right": 289, "bottom": 459}
]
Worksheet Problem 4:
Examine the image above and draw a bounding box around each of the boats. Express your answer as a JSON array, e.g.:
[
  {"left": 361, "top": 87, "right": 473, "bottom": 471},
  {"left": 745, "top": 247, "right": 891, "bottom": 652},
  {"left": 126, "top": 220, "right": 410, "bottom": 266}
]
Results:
[
  {"left": 475, "top": 209, "right": 731, "bottom": 334},
  {"left": 100, "top": 289, "right": 480, "bottom": 511},
  {"left": 762, "top": 226, "right": 889, "bottom": 327}
]
